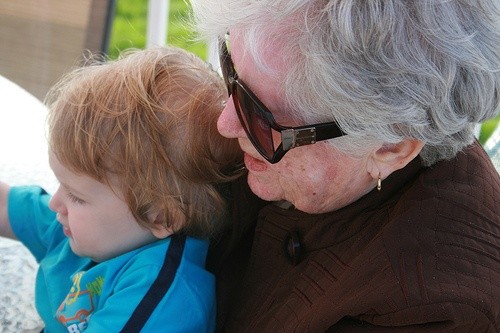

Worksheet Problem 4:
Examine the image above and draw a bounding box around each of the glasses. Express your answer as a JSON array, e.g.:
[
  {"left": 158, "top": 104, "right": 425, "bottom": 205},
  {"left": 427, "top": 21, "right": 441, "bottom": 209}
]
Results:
[{"left": 217, "top": 32, "right": 363, "bottom": 165}]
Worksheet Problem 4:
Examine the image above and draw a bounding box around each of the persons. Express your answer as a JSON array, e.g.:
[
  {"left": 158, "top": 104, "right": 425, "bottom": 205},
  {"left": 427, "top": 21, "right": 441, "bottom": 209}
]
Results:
[
  {"left": 180, "top": 0, "right": 500, "bottom": 333},
  {"left": 1, "top": 41, "right": 242, "bottom": 332}
]
